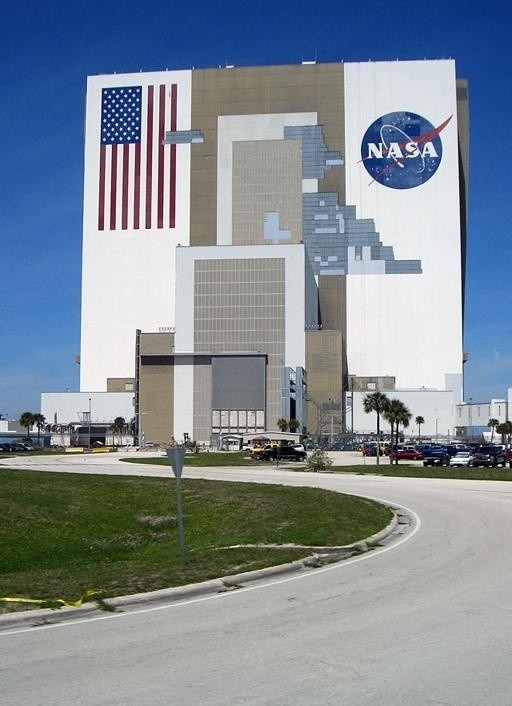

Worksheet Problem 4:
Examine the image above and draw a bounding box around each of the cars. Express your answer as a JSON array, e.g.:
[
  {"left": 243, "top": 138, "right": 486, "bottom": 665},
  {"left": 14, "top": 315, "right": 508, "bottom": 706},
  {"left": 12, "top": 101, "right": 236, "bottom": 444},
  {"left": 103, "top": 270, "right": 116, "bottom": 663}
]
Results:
[
  {"left": 362, "top": 440, "right": 415, "bottom": 456},
  {"left": 242, "top": 442, "right": 307, "bottom": 461},
  {"left": 0, "top": 442, "right": 36, "bottom": 452},
  {"left": 415, "top": 442, "right": 476, "bottom": 456},
  {"left": 391, "top": 450, "right": 422, "bottom": 461},
  {"left": 422, "top": 452, "right": 450, "bottom": 468},
  {"left": 449, "top": 451, "right": 475, "bottom": 468}
]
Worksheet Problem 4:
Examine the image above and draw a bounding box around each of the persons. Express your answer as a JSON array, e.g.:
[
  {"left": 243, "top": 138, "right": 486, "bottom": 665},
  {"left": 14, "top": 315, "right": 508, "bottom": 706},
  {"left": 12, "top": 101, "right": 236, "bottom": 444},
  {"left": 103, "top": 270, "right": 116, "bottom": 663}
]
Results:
[{"left": 503, "top": 446, "right": 512, "bottom": 470}]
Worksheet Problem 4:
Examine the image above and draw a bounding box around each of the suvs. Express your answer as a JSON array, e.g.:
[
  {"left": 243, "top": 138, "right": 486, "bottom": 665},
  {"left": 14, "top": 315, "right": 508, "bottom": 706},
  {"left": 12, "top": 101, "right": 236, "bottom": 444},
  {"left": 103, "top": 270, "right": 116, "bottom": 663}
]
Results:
[{"left": 472, "top": 445, "right": 505, "bottom": 468}]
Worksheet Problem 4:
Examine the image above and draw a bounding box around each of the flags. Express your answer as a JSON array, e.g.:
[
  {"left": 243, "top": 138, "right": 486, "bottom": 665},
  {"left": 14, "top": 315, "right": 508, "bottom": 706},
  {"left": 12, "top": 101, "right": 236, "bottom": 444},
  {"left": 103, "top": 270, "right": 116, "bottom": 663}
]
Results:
[{"left": 98, "top": 80, "right": 176, "bottom": 231}]
